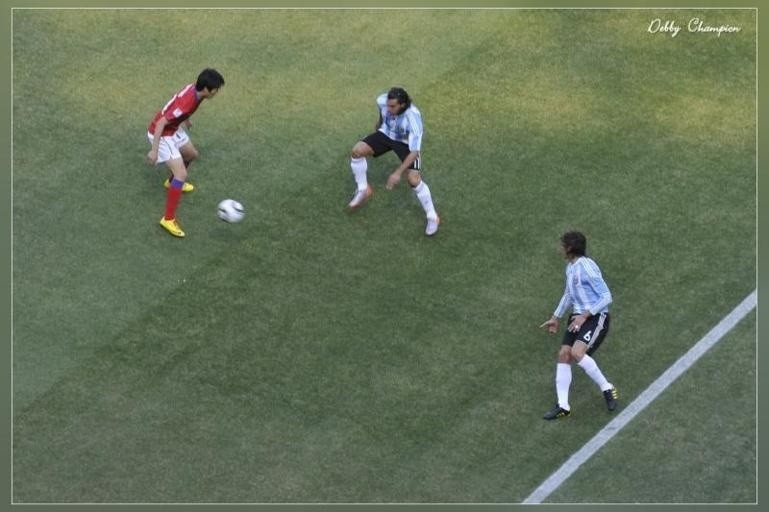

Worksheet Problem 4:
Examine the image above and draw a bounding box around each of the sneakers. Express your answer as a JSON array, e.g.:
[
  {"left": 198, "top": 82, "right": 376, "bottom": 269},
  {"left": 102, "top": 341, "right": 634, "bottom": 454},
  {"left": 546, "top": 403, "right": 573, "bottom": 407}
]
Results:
[
  {"left": 602, "top": 384, "right": 619, "bottom": 412},
  {"left": 541, "top": 404, "right": 572, "bottom": 421},
  {"left": 159, "top": 216, "right": 186, "bottom": 238},
  {"left": 163, "top": 179, "right": 194, "bottom": 192},
  {"left": 348, "top": 183, "right": 373, "bottom": 210},
  {"left": 424, "top": 212, "right": 440, "bottom": 236}
]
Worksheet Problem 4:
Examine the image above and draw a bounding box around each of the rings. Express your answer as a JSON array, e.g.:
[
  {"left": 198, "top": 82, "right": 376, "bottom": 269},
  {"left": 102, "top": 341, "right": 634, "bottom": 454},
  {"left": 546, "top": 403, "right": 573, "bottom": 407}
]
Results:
[{"left": 575, "top": 324, "right": 578, "bottom": 328}]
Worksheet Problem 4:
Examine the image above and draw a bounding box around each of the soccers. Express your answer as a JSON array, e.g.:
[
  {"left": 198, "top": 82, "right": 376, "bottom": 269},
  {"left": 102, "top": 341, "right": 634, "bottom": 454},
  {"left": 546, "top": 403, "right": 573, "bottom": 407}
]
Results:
[{"left": 217, "top": 199, "right": 245, "bottom": 223}]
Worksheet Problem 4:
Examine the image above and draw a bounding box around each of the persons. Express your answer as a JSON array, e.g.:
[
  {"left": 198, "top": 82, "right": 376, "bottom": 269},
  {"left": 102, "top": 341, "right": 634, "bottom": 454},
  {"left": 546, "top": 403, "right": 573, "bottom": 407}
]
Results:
[
  {"left": 538, "top": 230, "right": 621, "bottom": 420},
  {"left": 344, "top": 86, "right": 442, "bottom": 236},
  {"left": 146, "top": 67, "right": 225, "bottom": 237}
]
